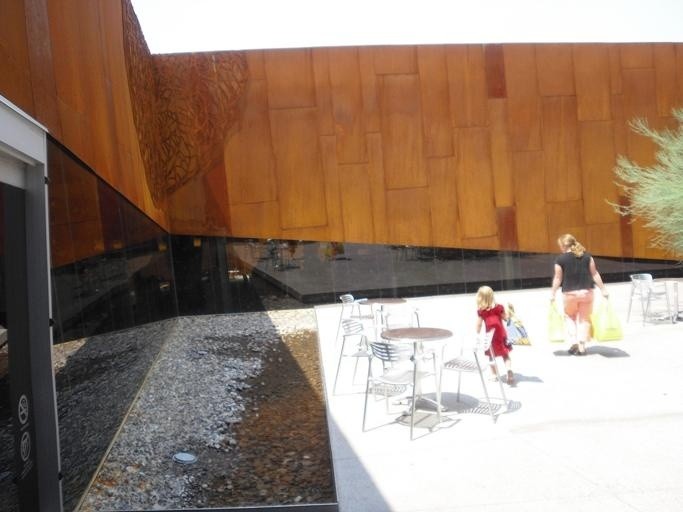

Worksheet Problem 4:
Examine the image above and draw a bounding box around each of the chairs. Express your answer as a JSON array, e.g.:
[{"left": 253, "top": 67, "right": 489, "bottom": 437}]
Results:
[
  {"left": 628, "top": 274, "right": 679, "bottom": 324},
  {"left": 335, "top": 294, "right": 508, "bottom": 441}
]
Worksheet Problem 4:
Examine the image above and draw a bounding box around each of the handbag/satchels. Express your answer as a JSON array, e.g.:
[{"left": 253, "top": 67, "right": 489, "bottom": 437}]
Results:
[{"left": 504, "top": 319, "right": 530, "bottom": 346}]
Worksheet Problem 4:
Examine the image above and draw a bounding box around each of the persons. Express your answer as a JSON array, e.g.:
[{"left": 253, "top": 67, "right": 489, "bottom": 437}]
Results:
[
  {"left": 549, "top": 233, "right": 609, "bottom": 356},
  {"left": 324, "top": 242, "right": 338, "bottom": 264},
  {"left": 285, "top": 239, "right": 298, "bottom": 260},
  {"left": 475, "top": 285, "right": 515, "bottom": 386}
]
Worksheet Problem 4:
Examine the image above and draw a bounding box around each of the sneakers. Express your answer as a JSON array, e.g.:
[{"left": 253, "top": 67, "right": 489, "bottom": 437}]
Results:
[
  {"left": 508, "top": 369, "right": 515, "bottom": 386},
  {"left": 579, "top": 351, "right": 585, "bottom": 356},
  {"left": 569, "top": 344, "right": 579, "bottom": 354}
]
